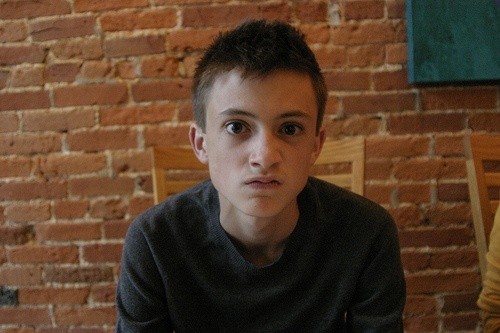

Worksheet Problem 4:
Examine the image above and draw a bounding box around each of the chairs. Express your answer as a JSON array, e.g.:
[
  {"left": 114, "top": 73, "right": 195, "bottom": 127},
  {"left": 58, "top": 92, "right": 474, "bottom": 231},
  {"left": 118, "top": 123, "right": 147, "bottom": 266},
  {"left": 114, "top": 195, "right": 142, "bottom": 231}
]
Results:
[
  {"left": 151, "top": 136, "right": 364, "bottom": 208},
  {"left": 460, "top": 130, "right": 500, "bottom": 292}
]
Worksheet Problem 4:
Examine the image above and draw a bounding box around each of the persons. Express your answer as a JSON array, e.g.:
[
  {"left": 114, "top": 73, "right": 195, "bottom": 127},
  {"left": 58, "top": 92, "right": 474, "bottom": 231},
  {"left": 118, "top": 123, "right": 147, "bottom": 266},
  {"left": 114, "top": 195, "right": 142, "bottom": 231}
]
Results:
[{"left": 115, "top": 18, "right": 408, "bottom": 332}]
